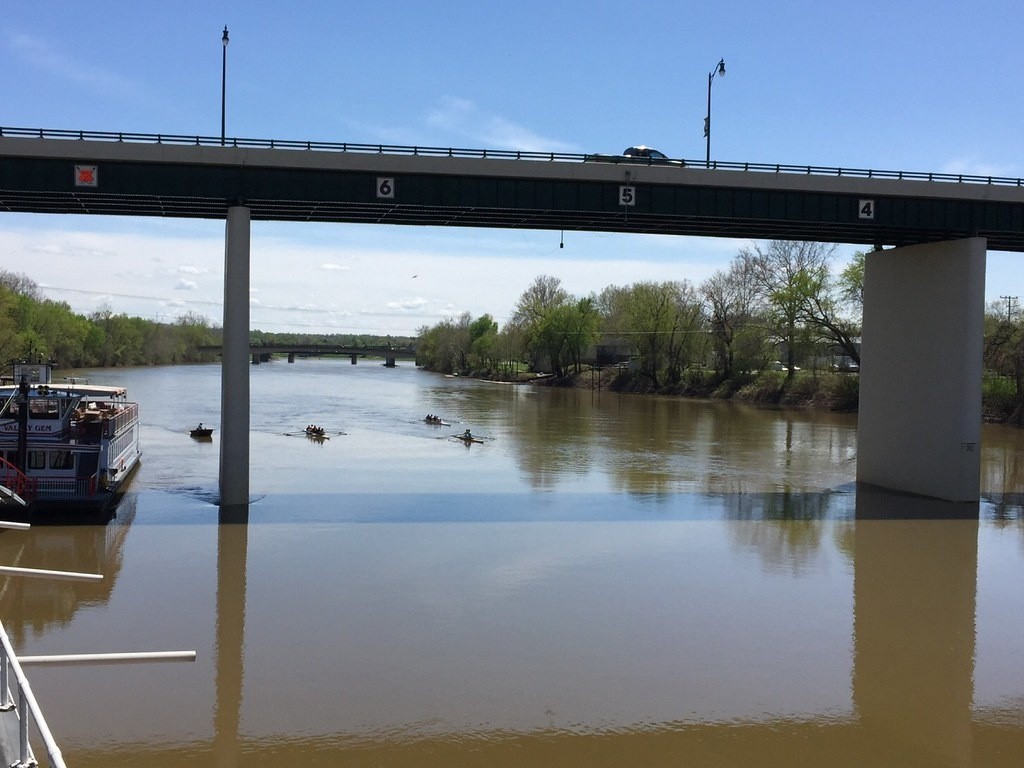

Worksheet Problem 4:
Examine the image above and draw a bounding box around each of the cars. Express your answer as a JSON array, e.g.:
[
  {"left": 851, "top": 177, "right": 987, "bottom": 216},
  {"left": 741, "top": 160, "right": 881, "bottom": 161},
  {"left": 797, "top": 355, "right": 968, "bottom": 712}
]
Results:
[
  {"left": 829, "top": 363, "right": 859, "bottom": 372},
  {"left": 781, "top": 365, "right": 801, "bottom": 371}
]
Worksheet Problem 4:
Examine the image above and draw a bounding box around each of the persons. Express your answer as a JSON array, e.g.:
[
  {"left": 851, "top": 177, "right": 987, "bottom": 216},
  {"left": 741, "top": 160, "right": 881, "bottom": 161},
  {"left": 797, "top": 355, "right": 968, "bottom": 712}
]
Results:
[
  {"left": 198, "top": 423, "right": 203, "bottom": 429},
  {"left": 465, "top": 429, "right": 472, "bottom": 438},
  {"left": 306, "top": 424, "right": 324, "bottom": 435},
  {"left": 426, "top": 414, "right": 439, "bottom": 421}
]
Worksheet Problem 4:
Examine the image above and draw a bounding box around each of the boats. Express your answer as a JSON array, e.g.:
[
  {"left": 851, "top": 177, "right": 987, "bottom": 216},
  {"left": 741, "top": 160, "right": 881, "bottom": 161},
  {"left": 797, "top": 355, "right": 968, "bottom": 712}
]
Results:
[
  {"left": 423, "top": 419, "right": 451, "bottom": 426},
  {"left": 452, "top": 435, "right": 486, "bottom": 443},
  {"left": 190, "top": 428, "right": 213, "bottom": 437},
  {"left": 0, "top": 339, "right": 143, "bottom": 515},
  {"left": 303, "top": 429, "right": 330, "bottom": 440}
]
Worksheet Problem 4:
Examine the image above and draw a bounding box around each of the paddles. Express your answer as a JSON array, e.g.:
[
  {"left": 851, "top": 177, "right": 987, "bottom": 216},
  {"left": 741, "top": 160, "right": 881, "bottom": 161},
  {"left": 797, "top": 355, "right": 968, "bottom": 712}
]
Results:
[
  {"left": 406, "top": 419, "right": 430, "bottom": 424},
  {"left": 324, "top": 431, "right": 348, "bottom": 436},
  {"left": 471, "top": 434, "right": 497, "bottom": 441},
  {"left": 439, "top": 418, "right": 462, "bottom": 424},
  {"left": 283, "top": 430, "right": 309, "bottom": 437},
  {"left": 434, "top": 433, "right": 466, "bottom": 441}
]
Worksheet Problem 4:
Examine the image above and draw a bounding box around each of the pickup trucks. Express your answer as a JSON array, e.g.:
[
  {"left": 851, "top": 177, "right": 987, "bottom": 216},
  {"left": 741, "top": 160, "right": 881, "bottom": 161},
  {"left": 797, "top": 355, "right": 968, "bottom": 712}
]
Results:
[{"left": 584, "top": 147, "right": 688, "bottom": 167}]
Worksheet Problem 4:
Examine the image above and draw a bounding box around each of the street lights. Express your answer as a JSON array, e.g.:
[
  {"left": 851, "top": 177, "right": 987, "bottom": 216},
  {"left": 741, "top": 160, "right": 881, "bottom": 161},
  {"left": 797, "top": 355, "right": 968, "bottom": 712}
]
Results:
[
  {"left": 706, "top": 58, "right": 726, "bottom": 168},
  {"left": 221, "top": 24, "right": 229, "bottom": 146}
]
